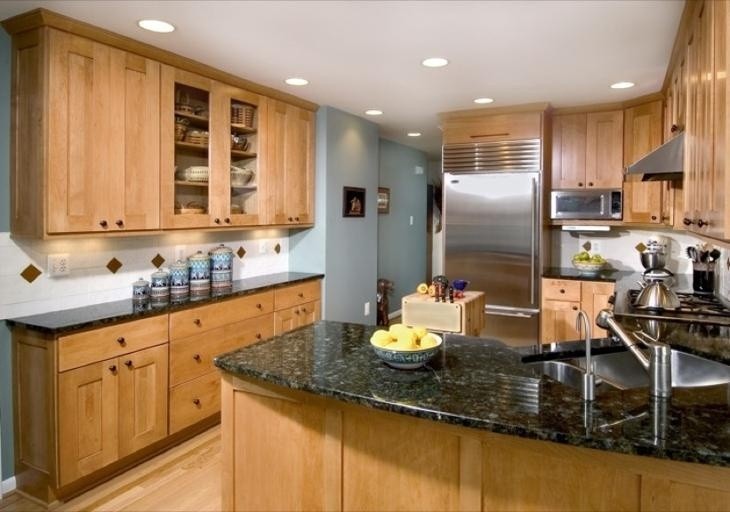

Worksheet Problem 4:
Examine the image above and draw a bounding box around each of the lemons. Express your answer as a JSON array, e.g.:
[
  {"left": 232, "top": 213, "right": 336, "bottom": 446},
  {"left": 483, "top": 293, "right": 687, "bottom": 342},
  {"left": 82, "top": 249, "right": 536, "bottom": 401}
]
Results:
[{"left": 372, "top": 322, "right": 436, "bottom": 351}]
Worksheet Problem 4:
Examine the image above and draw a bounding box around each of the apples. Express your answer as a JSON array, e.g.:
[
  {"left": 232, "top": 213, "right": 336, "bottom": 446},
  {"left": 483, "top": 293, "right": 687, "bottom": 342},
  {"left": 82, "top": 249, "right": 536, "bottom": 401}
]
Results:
[{"left": 573, "top": 252, "right": 606, "bottom": 264}]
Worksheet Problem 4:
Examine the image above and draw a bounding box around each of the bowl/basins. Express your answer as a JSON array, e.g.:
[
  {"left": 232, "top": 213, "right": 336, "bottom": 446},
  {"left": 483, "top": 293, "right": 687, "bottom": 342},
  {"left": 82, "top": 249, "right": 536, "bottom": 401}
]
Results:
[
  {"left": 231, "top": 171, "right": 252, "bottom": 185},
  {"left": 185, "top": 166, "right": 209, "bottom": 183},
  {"left": 369, "top": 331, "right": 443, "bottom": 370},
  {"left": 641, "top": 252, "right": 665, "bottom": 272},
  {"left": 175, "top": 208, "right": 205, "bottom": 214},
  {"left": 452, "top": 280, "right": 467, "bottom": 290},
  {"left": 573, "top": 262, "right": 606, "bottom": 276}
]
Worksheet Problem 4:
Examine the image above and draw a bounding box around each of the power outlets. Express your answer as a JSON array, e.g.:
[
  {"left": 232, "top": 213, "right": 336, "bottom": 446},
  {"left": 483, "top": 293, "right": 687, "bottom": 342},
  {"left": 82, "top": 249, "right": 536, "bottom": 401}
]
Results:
[{"left": 47, "top": 252, "right": 72, "bottom": 278}]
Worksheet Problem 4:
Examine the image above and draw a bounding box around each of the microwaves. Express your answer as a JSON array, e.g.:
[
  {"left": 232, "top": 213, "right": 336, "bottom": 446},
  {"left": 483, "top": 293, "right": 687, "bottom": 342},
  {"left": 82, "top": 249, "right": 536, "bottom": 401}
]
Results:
[{"left": 551, "top": 188, "right": 623, "bottom": 220}]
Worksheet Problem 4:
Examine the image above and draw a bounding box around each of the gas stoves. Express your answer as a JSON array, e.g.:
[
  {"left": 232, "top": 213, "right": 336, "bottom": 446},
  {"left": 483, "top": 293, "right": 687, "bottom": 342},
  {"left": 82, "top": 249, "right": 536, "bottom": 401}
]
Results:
[
  {"left": 614, "top": 288, "right": 730, "bottom": 325},
  {"left": 621, "top": 131, "right": 685, "bottom": 184}
]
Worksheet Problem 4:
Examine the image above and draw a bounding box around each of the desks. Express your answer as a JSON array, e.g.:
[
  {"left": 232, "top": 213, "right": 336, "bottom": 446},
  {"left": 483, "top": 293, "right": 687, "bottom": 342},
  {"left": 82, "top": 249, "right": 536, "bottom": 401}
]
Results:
[{"left": 400, "top": 289, "right": 486, "bottom": 337}]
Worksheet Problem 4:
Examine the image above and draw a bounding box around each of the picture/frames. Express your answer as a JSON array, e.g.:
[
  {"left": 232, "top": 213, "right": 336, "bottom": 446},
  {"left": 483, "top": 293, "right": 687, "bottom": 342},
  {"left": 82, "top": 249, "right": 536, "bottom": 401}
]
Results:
[
  {"left": 377, "top": 187, "right": 391, "bottom": 214},
  {"left": 343, "top": 185, "right": 365, "bottom": 219}
]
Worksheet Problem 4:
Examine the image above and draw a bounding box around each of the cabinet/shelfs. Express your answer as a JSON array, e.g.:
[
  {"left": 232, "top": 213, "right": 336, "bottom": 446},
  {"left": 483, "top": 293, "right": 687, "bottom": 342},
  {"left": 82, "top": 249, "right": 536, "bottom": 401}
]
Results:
[
  {"left": 549, "top": 110, "right": 625, "bottom": 189},
  {"left": 624, "top": 101, "right": 673, "bottom": 227},
  {"left": 669, "top": 47, "right": 686, "bottom": 135},
  {"left": 443, "top": 109, "right": 550, "bottom": 144},
  {"left": 681, "top": 1, "right": 730, "bottom": 244},
  {"left": 276, "top": 278, "right": 323, "bottom": 338},
  {"left": 8, "top": 314, "right": 170, "bottom": 489},
  {"left": 542, "top": 279, "right": 583, "bottom": 345},
  {"left": 169, "top": 291, "right": 275, "bottom": 435},
  {"left": 585, "top": 282, "right": 615, "bottom": 339}
]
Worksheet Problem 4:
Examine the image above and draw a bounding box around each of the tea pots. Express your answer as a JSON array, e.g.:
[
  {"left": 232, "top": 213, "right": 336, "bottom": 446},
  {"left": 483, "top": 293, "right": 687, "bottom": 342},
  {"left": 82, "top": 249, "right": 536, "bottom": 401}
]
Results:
[{"left": 633, "top": 267, "right": 683, "bottom": 308}]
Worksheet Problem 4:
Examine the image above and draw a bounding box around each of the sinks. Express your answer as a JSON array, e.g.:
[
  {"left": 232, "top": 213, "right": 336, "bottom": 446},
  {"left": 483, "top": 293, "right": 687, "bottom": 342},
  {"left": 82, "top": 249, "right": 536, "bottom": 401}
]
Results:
[
  {"left": 522, "top": 360, "right": 622, "bottom": 391},
  {"left": 568, "top": 349, "right": 730, "bottom": 388}
]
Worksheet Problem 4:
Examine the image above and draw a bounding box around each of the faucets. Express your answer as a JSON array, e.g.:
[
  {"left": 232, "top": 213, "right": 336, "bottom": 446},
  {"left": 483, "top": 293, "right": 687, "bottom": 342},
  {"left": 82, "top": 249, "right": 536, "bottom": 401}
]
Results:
[
  {"left": 574, "top": 310, "right": 594, "bottom": 402},
  {"left": 594, "top": 308, "right": 673, "bottom": 400}
]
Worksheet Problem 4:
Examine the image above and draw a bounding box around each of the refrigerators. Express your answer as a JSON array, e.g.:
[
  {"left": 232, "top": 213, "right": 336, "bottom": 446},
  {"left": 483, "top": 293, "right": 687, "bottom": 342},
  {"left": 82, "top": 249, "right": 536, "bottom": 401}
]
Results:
[{"left": 443, "top": 174, "right": 541, "bottom": 346}]
